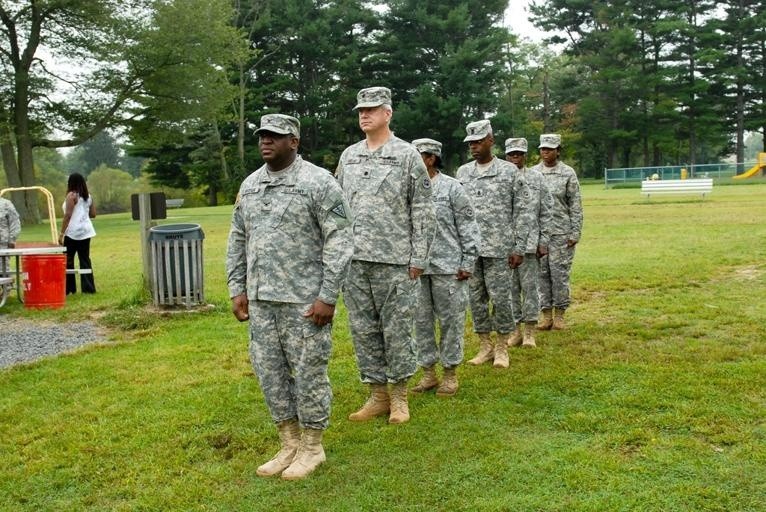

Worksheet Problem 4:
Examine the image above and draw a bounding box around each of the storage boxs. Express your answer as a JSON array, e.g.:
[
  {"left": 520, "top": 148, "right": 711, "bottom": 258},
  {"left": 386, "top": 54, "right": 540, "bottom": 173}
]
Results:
[
  {"left": 0, "top": 268, "right": 92, "bottom": 276},
  {"left": 640, "top": 178, "right": 713, "bottom": 198}
]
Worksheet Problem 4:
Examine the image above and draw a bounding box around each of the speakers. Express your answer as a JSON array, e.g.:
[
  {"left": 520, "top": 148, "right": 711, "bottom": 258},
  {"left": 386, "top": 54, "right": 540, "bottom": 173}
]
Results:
[
  {"left": 388, "top": 380, "right": 410, "bottom": 423},
  {"left": 349, "top": 384, "right": 390, "bottom": 421},
  {"left": 537, "top": 310, "right": 553, "bottom": 329},
  {"left": 435, "top": 364, "right": 459, "bottom": 397},
  {"left": 281, "top": 428, "right": 326, "bottom": 480},
  {"left": 255, "top": 420, "right": 302, "bottom": 477},
  {"left": 523, "top": 323, "right": 536, "bottom": 348},
  {"left": 553, "top": 308, "right": 565, "bottom": 329},
  {"left": 508, "top": 325, "right": 522, "bottom": 346},
  {"left": 411, "top": 366, "right": 439, "bottom": 394},
  {"left": 493, "top": 333, "right": 510, "bottom": 368},
  {"left": 466, "top": 332, "right": 494, "bottom": 366}
]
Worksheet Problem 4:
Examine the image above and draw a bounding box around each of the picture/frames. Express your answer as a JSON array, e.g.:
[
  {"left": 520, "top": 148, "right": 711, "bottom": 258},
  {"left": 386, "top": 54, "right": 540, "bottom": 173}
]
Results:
[{"left": 0, "top": 246, "right": 67, "bottom": 304}]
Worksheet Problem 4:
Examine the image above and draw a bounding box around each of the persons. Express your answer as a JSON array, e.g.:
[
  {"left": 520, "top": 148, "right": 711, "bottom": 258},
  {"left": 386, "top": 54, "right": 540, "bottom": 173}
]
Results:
[
  {"left": 332, "top": 86, "right": 433, "bottom": 425},
  {"left": 504, "top": 137, "right": 555, "bottom": 348},
  {"left": 409, "top": 138, "right": 481, "bottom": 397},
  {"left": 530, "top": 133, "right": 583, "bottom": 331},
  {"left": 58, "top": 172, "right": 97, "bottom": 295},
  {"left": 0, "top": 188, "right": 21, "bottom": 300},
  {"left": 454, "top": 120, "right": 532, "bottom": 369},
  {"left": 224, "top": 114, "right": 355, "bottom": 481}
]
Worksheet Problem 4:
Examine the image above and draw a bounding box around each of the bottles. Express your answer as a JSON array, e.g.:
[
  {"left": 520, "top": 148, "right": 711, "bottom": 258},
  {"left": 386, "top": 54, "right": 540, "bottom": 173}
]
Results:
[
  {"left": 411, "top": 138, "right": 442, "bottom": 156},
  {"left": 504, "top": 138, "right": 528, "bottom": 154},
  {"left": 253, "top": 113, "right": 301, "bottom": 138},
  {"left": 463, "top": 120, "right": 492, "bottom": 142},
  {"left": 351, "top": 87, "right": 391, "bottom": 111},
  {"left": 538, "top": 134, "right": 561, "bottom": 149}
]
